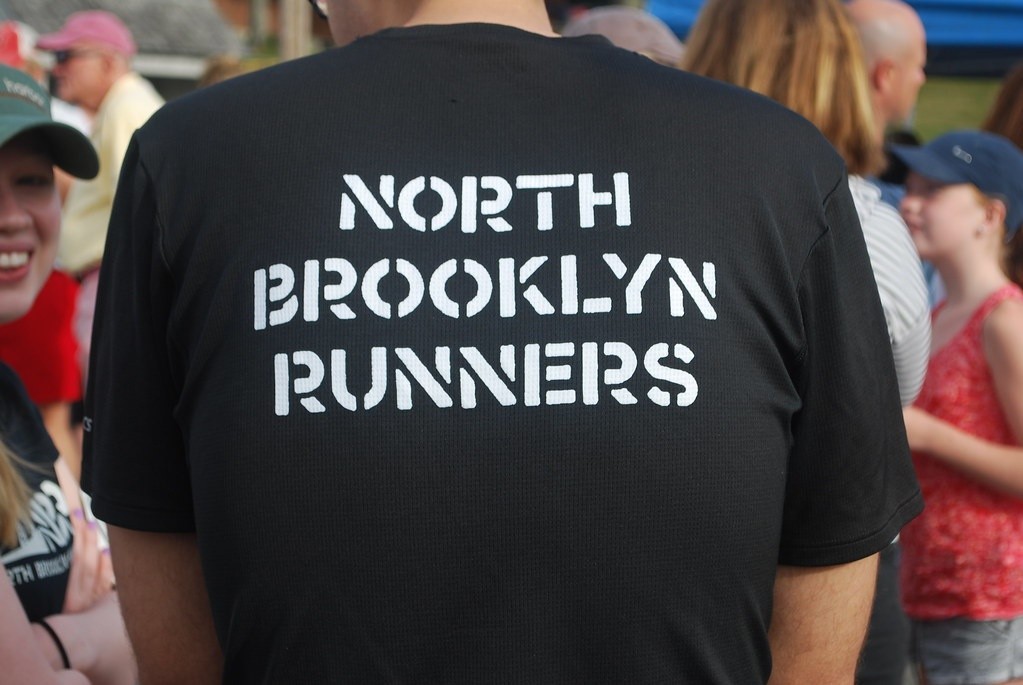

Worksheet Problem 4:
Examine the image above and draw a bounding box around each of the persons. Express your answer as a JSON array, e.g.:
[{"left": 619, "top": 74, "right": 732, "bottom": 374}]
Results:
[
  {"left": 78, "top": 0, "right": 926, "bottom": 685},
  {"left": 544, "top": 0, "right": 1023, "bottom": 685},
  {"left": 0, "top": 0, "right": 332, "bottom": 685}
]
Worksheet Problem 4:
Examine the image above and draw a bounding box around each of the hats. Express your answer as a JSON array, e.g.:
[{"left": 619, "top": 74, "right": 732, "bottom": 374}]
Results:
[
  {"left": 0, "top": 19, "right": 58, "bottom": 70},
  {"left": 37, "top": 7, "right": 135, "bottom": 57},
  {"left": 0, "top": 65, "right": 99, "bottom": 181},
  {"left": 885, "top": 129, "right": 1023, "bottom": 232}
]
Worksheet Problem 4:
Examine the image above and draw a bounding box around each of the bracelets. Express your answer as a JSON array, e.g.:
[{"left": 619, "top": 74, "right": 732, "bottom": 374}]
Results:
[{"left": 35, "top": 618, "right": 71, "bottom": 670}]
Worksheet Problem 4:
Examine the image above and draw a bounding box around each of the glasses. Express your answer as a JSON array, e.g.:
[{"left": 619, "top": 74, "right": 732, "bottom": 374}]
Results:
[{"left": 54, "top": 50, "right": 110, "bottom": 66}]
